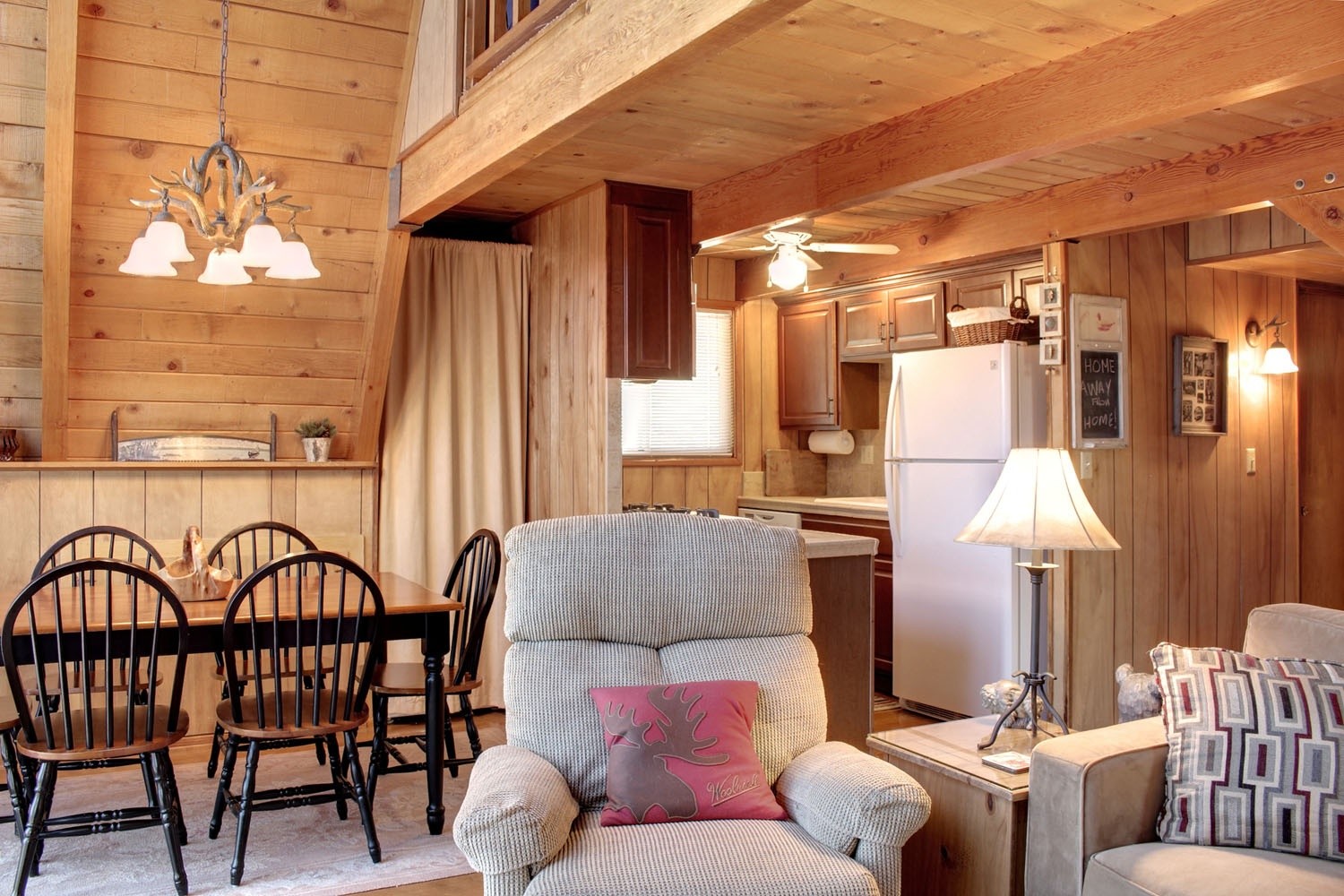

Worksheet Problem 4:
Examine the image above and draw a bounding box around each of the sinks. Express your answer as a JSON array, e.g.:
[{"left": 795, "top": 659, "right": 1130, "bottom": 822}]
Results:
[{"left": 814, "top": 495, "right": 887, "bottom": 508}]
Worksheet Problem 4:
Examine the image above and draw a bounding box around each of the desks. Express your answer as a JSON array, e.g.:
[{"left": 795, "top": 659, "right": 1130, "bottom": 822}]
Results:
[{"left": 0, "top": 569, "right": 467, "bottom": 838}]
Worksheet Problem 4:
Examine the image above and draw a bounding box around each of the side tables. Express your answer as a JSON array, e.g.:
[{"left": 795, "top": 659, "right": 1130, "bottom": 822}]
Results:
[{"left": 863, "top": 713, "right": 1087, "bottom": 896}]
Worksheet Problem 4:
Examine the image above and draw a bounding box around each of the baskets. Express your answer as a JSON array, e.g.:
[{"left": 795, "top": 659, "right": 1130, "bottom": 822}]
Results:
[{"left": 948, "top": 296, "right": 1030, "bottom": 346}]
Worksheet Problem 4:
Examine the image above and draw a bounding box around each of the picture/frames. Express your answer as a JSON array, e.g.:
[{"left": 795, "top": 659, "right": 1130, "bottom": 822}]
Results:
[{"left": 1039, "top": 280, "right": 1063, "bottom": 367}]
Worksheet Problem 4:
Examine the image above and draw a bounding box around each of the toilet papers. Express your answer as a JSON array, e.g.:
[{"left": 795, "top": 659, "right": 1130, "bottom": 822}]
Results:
[{"left": 807, "top": 430, "right": 855, "bottom": 455}]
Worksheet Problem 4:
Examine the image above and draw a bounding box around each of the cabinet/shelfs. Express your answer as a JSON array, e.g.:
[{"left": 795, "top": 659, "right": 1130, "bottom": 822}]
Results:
[
  {"left": 601, "top": 182, "right": 697, "bottom": 384},
  {"left": 770, "top": 255, "right": 1045, "bottom": 434}
]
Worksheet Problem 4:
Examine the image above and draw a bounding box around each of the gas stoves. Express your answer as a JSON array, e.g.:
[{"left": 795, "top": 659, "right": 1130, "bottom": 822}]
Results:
[{"left": 622, "top": 500, "right": 749, "bottom": 520}]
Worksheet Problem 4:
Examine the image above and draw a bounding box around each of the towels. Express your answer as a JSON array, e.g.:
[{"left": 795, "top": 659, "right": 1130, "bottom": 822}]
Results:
[{"left": 947, "top": 306, "right": 1011, "bottom": 327}]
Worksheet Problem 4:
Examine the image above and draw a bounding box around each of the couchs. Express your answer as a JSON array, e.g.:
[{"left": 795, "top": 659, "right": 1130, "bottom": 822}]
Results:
[
  {"left": 451, "top": 511, "right": 931, "bottom": 896},
  {"left": 1021, "top": 604, "right": 1344, "bottom": 896}
]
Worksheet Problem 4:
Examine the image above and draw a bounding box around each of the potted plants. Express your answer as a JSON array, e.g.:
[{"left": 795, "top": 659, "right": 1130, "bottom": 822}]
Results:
[{"left": 293, "top": 418, "right": 338, "bottom": 462}]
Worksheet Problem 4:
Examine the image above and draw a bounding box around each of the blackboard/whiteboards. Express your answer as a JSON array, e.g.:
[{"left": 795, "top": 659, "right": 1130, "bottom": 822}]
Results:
[{"left": 1070, "top": 293, "right": 1129, "bottom": 449}]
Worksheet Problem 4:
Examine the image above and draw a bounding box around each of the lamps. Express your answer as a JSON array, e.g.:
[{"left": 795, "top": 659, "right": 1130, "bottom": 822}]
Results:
[
  {"left": 767, "top": 243, "right": 809, "bottom": 292},
  {"left": 1245, "top": 315, "right": 1299, "bottom": 377},
  {"left": 118, "top": 0, "right": 321, "bottom": 283},
  {"left": 946, "top": 446, "right": 1121, "bottom": 749}
]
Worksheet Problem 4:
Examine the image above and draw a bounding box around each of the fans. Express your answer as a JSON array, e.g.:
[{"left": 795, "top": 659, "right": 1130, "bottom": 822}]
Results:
[{"left": 695, "top": 223, "right": 901, "bottom": 272}]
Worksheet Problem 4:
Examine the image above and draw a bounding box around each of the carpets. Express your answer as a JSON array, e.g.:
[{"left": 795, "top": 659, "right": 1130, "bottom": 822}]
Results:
[{"left": 0, "top": 721, "right": 508, "bottom": 896}]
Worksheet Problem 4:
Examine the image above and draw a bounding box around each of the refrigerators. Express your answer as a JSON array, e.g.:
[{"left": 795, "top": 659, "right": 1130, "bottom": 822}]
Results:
[{"left": 883, "top": 338, "right": 1049, "bottom": 719}]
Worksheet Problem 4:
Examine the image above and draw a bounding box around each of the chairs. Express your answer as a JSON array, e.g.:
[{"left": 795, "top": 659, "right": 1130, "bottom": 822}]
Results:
[
  {"left": 206, "top": 551, "right": 387, "bottom": 886},
  {"left": 1, "top": 557, "right": 190, "bottom": 896},
  {"left": 360, "top": 526, "right": 502, "bottom": 827},
  {"left": 0, "top": 517, "right": 327, "bottom": 833}
]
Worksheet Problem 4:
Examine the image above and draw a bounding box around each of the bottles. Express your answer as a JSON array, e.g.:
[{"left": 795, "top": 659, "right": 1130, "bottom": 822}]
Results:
[{"left": 0, "top": 429, "right": 19, "bottom": 462}]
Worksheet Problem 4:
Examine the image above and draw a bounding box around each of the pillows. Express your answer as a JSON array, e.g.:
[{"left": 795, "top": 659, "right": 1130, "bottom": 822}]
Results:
[
  {"left": 1150, "top": 639, "right": 1343, "bottom": 862},
  {"left": 587, "top": 680, "right": 790, "bottom": 825}
]
